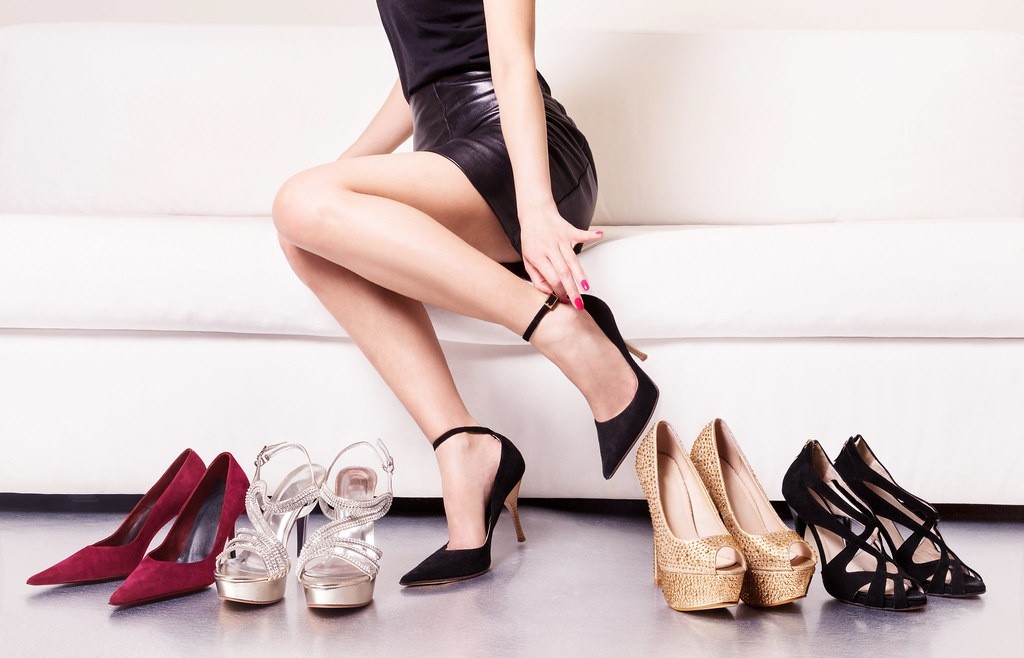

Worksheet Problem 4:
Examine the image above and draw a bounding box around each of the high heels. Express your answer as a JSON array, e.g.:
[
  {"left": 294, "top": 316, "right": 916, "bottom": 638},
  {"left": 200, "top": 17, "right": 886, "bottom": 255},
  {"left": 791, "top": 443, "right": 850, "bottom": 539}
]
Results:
[
  {"left": 783, "top": 438, "right": 929, "bottom": 611},
  {"left": 634, "top": 420, "right": 748, "bottom": 612},
  {"left": 26, "top": 448, "right": 207, "bottom": 586},
  {"left": 834, "top": 434, "right": 986, "bottom": 598},
  {"left": 399, "top": 426, "right": 525, "bottom": 586},
  {"left": 213, "top": 442, "right": 328, "bottom": 604},
  {"left": 691, "top": 418, "right": 819, "bottom": 608},
  {"left": 522, "top": 288, "right": 660, "bottom": 479},
  {"left": 296, "top": 436, "right": 393, "bottom": 609},
  {"left": 109, "top": 451, "right": 250, "bottom": 606}
]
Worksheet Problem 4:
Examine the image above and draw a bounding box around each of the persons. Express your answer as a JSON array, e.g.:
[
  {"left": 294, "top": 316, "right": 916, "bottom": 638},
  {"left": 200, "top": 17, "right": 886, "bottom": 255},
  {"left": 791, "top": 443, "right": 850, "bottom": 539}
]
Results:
[{"left": 273, "top": 0, "right": 659, "bottom": 586}]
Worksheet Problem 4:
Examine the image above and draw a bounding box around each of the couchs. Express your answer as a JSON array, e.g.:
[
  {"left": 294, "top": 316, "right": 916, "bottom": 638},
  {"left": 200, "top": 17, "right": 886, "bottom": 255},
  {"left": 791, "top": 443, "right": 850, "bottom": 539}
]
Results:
[{"left": 0, "top": 21, "right": 1022, "bottom": 506}]
